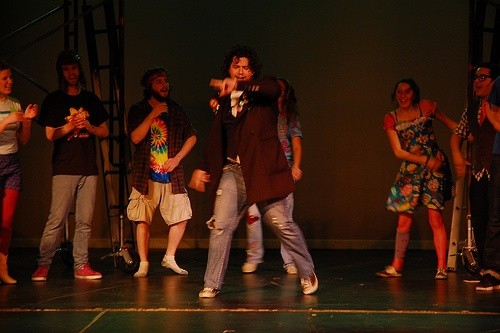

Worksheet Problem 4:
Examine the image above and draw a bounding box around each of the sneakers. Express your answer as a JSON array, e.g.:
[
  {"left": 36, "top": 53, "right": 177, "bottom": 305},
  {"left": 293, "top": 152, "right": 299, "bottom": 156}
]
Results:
[
  {"left": 31, "top": 265, "right": 50, "bottom": 281},
  {"left": 74, "top": 262, "right": 102, "bottom": 279}
]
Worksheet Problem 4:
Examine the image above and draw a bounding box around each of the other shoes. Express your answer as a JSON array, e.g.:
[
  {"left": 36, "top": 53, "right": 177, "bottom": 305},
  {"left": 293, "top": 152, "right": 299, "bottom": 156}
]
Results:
[
  {"left": 134, "top": 261, "right": 150, "bottom": 278},
  {"left": 376, "top": 265, "right": 402, "bottom": 277},
  {"left": 161, "top": 254, "right": 188, "bottom": 275},
  {"left": 285, "top": 265, "right": 298, "bottom": 275},
  {"left": 463, "top": 270, "right": 483, "bottom": 282},
  {"left": 300, "top": 270, "right": 318, "bottom": 295},
  {"left": 199, "top": 287, "right": 220, "bottom": 298},
  {"left": 474, "top": 273, "right": 498, "bottom": 289},
  {"left": 435, "top": 267, "right": 448, "bottom": 279},
  {"left": 242, "top": 262, "right": 262, "bottom": 273}
]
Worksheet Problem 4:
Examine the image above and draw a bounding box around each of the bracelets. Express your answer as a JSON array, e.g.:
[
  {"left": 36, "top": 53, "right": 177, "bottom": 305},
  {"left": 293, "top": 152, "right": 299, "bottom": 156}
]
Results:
[
  {"left": 92, "top": 125, "right": 95, "bottom": 134},
  {"left": 431, "top": 158, "right": 438, "bottom": 170},
  {"left": 424, "top": 155, "right": 430, "bottom": 167}
]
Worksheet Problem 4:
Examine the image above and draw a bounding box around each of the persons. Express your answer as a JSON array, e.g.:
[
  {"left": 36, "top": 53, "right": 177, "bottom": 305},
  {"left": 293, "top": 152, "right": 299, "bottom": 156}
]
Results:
[
  {"left": 31, "top": 51, "right": 110, "bottom": 282},
  {"left": 449, "top": 63, "right": 500, "bottom": 291},
  {"left": 126, "top": 67, "right": 198, "bottom": 278},
  {"left": 0, "top": 63, "right": 38, "bottom": 285},
  {"left": 187, "top": 40, "right": 319, "bottom": 297},
  {"left": 375, "top": 77, "right": 474, "bottom": 280},
  {"left": 240, "top": 75, "right": 304, "bottom": 274}
]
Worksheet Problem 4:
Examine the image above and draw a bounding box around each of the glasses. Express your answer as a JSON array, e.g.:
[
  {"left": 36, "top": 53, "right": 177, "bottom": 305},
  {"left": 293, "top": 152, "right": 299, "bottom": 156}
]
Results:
[{"left": 473, "top": 73, "right": 491, "bottom": 81}]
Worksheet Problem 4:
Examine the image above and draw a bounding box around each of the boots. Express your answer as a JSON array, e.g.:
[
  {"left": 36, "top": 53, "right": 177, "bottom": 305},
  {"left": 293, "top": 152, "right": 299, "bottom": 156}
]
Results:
[{"left": 0, "top": 252, "right": 17, "bottom": 285}]
